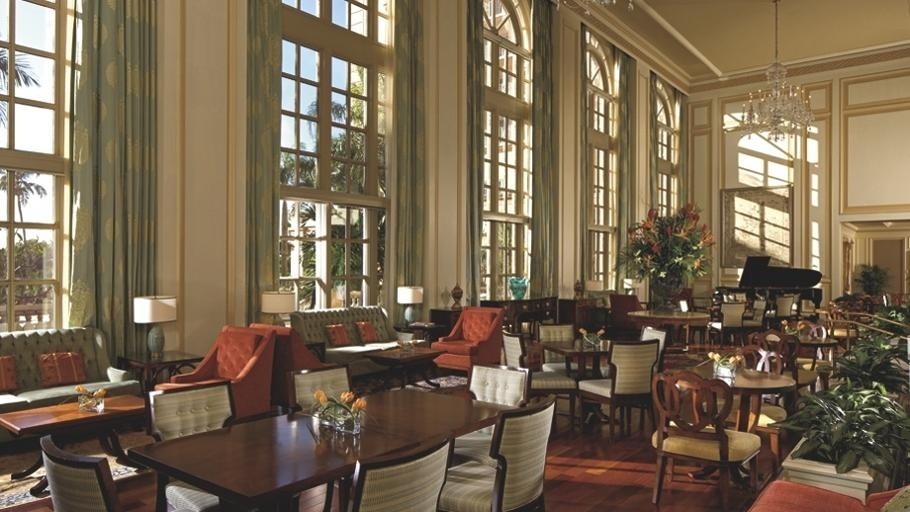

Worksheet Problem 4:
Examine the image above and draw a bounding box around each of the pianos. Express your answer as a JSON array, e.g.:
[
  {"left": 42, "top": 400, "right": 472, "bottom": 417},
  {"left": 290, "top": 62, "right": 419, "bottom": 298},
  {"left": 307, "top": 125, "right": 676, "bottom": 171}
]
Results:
[{"left": 740, "top": 257, "right": 822, "bottom": 287}]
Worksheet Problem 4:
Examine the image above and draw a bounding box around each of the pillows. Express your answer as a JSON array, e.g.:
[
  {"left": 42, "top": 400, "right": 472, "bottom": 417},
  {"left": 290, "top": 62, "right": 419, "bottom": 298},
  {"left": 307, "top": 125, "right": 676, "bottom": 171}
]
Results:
[
  {"left": 0, "top": 355, "right": 20, "bottom": 391},
  {"left": 39, "top": 350, "right": 88, "bottom": 386}
]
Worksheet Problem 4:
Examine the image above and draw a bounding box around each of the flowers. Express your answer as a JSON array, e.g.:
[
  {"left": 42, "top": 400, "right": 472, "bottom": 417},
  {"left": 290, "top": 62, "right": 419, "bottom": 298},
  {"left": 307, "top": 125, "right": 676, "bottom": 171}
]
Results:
[
  {"left": 75, "top": 384, "right": 107, "bottom": 407},
  {"left": 614, "top": 201, "right": 717, "bottom": 301},
  {"left": 312, "top": 387, "right": 369, "bottom": 415}
]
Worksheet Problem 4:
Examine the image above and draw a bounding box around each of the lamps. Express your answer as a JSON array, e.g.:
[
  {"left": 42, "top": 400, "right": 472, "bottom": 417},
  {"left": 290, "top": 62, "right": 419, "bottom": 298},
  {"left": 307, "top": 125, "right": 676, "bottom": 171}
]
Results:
[
  {"left": 260, "top": 290, "right": 296, "bottom": 325},
  {"left": 396, "top": 286, "right": 424, "bottom": 323},
  {"left": 133, "top": 295, "right": 178, "bottom": 358},
  {"left": 740, "top": 0, "right": 813, "bottom": 142}
]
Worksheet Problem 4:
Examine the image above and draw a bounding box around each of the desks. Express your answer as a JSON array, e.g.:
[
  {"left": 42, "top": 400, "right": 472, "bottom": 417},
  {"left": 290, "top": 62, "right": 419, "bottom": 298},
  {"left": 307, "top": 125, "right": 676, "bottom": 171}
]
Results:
[
  {"left": 117, "top": 349, "right": 203, "bottom": 389},
  {"left": 364, "top": 345, "right": 441, "bottom": 391},
  {"left": 128, "top": 388, "right": 524, "bottom": 506}
]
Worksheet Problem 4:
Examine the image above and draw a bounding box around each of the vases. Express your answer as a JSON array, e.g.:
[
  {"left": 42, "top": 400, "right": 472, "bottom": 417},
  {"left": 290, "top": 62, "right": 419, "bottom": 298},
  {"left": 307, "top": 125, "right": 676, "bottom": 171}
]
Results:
[{"left": 78, "top": 394, "right": 105, "bottom": 412}]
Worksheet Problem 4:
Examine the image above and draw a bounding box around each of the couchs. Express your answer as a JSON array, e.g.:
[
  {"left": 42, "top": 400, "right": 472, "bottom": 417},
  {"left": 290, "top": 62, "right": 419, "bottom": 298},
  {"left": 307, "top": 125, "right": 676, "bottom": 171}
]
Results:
[{"left": 0, "top": 325, "right": 142, "bottom": 444}]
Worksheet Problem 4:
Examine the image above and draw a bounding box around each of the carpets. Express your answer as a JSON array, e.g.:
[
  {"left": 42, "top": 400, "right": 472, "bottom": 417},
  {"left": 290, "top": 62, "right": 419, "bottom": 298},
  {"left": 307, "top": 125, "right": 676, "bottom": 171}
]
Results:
[{"left": 0, "top": 428, "right": 160, "bottom": 509}]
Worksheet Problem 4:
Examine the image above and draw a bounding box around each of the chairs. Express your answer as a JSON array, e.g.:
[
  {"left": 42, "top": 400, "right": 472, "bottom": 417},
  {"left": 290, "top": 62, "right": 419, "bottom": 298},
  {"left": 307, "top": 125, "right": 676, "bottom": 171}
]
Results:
[
  {"left": 249, "top": 322, "right": 327, "bottom": 406},
  {"left": 431, "top": 306, "right": 503, "bottom": 375},
  {"left": 747, "top": 479, "right": 910, "bottom": 511},
  {"left": 649, "top": 369, "right": 762, "bottom": 510},
  {"left": 148, "top": 380, "right": 237, "bottom": 511},
  {"left": 345, "top": 431, "right": 456, "bottom": 511},
  {"left": 500, "top": 286, "right": 909, "bottom": 446},
  {"left": 171, "top": 321, "right": 277, "bottom": 421},
  {"left": 291, "top": 303, "right": 416, "bottom": 378},
  {"left": 287, "top": 364, "right": 352, "bottom": 415},
  {"left": 439, "top": 393, "right": 560, "bottom": 511},
  {"left": 464, "top": 360, "right": 531, "bottom": 411},
  {"left": 39, "top": 433, "right": 123, "bottom": 510}
]
